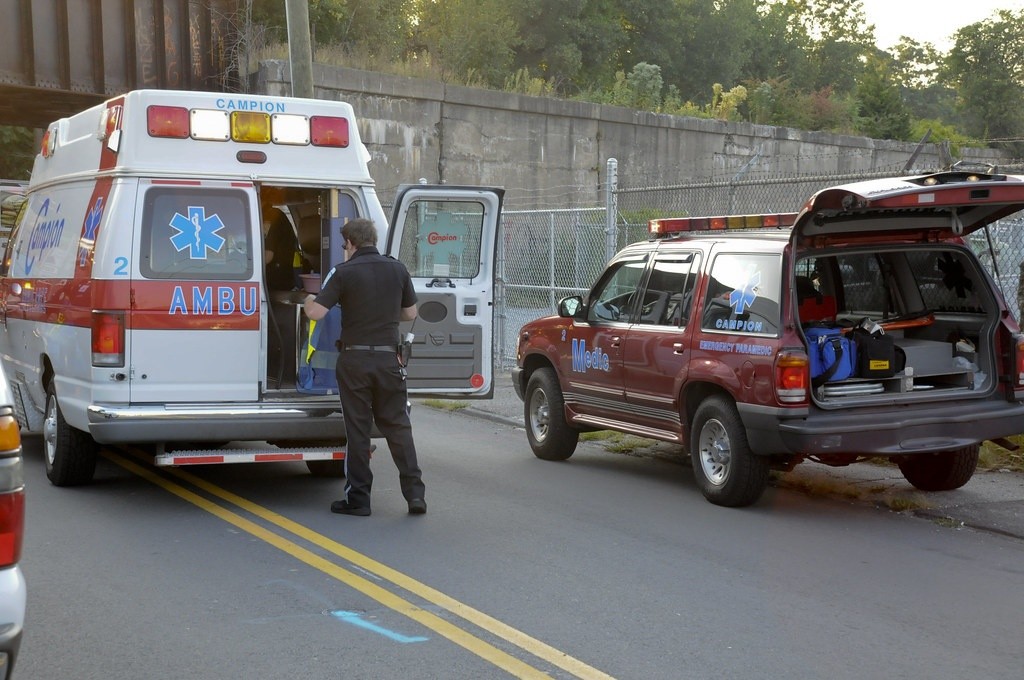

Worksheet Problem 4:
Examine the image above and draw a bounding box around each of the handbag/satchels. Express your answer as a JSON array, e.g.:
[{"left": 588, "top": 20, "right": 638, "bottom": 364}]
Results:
[
  {"left": 804, "top": 321, "right": 855, "bottom": 384},
  {"left": 846, "top": 323, "right": 906, "bottom": 377}
]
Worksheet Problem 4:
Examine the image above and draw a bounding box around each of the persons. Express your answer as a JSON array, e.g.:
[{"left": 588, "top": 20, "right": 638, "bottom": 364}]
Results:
[
  {"left": 303, "top": 217, "right": 428, "bottom": 517},
  {"left": 263, "top": 204, "right": 296, "bottom": 290}
]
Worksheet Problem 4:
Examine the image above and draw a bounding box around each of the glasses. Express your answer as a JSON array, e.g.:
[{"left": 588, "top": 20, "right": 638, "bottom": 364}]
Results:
[{"left": 342, "top": 243, "right": 347, "bottom": 249}]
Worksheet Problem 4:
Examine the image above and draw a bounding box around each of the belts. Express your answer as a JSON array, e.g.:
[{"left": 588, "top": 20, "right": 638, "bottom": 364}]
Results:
[{"left": 344, "top": 344, "right": 400, "bottom": 353}]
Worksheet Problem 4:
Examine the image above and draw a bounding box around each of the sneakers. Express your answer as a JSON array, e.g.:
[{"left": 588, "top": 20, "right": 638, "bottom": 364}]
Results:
[
  {"left": 407, "top": 498, "right": 428, "bottom": 514},
  {"left": 331, "top": 498, "right": 371, "bottom": 516}
]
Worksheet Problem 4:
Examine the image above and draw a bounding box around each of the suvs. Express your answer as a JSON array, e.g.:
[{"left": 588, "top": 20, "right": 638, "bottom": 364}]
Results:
[{"left": 509, "top": 158, "right": 1024, "bottom": 508}]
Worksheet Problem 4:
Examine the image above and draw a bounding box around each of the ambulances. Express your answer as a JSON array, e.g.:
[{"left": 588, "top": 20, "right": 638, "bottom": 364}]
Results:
[{"left": 0, "top": 89, "right": 508, "bottom": 489}]
